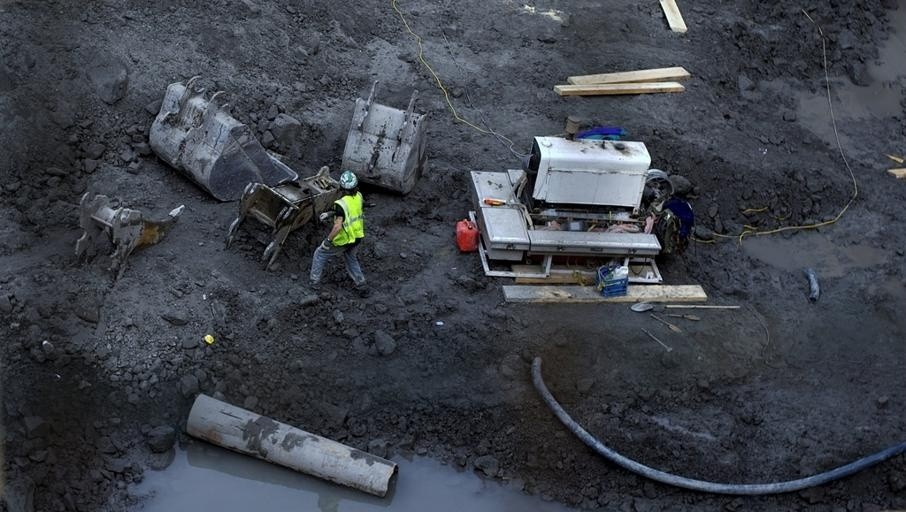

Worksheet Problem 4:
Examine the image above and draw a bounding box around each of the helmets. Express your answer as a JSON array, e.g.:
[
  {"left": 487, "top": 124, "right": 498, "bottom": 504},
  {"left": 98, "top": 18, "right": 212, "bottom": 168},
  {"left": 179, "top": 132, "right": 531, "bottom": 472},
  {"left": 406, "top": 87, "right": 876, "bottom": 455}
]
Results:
[{"left": 339, "top": 169, "right": 359, "bottom": 190}]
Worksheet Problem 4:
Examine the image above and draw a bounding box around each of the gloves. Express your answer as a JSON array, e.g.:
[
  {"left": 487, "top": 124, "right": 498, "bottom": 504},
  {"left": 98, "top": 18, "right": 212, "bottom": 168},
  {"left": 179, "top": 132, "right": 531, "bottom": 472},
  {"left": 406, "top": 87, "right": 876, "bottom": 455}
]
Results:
[
  {"left": 319, "top": 212, "right": 329, "bottom": 221},
  {"left": 318, "top": 239, "right": 332, "bottom": 253}
]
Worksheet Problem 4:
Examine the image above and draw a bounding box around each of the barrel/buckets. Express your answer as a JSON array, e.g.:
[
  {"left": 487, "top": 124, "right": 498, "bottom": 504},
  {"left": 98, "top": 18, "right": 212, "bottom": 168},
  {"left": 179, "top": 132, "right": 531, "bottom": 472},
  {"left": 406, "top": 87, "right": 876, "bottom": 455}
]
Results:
[
  {"left": 457, "top": 217, "right": 480, "bottom": 251},
  {"left": 614, "top": 263, "right": 630, "bottom": 279}
]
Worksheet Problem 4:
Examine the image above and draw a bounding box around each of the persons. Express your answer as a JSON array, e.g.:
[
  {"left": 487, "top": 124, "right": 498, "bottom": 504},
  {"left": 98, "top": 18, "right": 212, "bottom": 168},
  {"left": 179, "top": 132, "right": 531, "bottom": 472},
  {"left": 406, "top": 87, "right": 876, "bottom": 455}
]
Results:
[{"left": 307, "top": 169, "right": 374, "bottom": 298}]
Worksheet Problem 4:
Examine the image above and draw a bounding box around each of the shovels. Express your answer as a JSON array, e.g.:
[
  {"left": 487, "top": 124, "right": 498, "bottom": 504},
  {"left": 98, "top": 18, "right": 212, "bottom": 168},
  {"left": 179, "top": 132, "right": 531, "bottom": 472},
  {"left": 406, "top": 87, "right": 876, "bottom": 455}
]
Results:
[
  {"left": 631, "top": 303, "right": 741, "bottom": 311},
  {"left": 651, "top": 313, "right": 682, "bottom": 333},
  {"left": 666, "top": 314, "right": 700, "bottom": 321}
]
